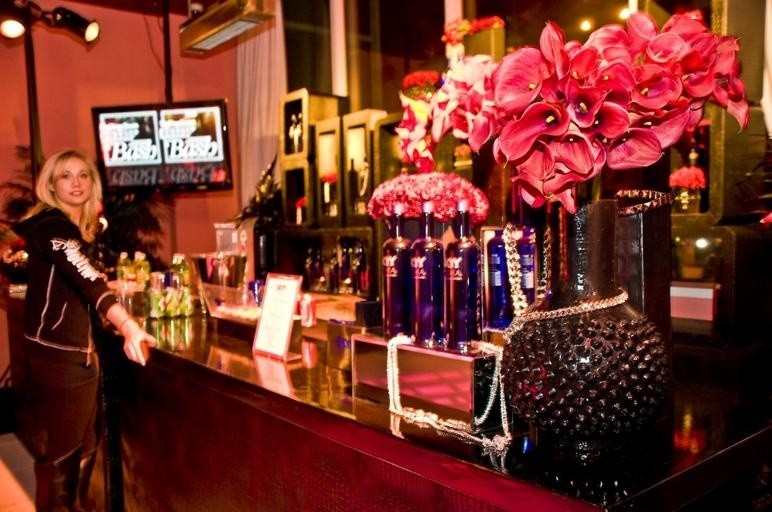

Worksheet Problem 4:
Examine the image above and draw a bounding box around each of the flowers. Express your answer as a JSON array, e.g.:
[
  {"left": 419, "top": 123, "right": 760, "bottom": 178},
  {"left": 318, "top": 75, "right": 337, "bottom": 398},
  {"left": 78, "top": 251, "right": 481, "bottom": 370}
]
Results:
[
  {"left": 489, "top": 8, "right": 751, "bottom": 216},
  {"left": 667, "top": 166, "right": 706, "bottom": 191},
  {"left": 396, "top": 53, "right": 501, "bottom": 174}
]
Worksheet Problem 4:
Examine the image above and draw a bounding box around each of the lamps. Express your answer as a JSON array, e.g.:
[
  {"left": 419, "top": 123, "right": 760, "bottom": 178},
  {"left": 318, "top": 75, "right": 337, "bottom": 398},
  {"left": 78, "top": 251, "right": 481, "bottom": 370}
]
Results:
[
  {"left": 0, "top": 0, "right": 32, "bottom": 39},
  {"left": 177, "top": 0, "right": 277, "bottom": 58},
  {"left": 51, "top": 6, "right": 100, "bottom": 43}
]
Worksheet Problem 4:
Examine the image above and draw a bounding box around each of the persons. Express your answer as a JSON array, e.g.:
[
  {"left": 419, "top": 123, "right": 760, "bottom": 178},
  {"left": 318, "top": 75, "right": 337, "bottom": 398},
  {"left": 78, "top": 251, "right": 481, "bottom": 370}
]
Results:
[{"left": 0, "top": 149, "right": 158, "bottom": 510}]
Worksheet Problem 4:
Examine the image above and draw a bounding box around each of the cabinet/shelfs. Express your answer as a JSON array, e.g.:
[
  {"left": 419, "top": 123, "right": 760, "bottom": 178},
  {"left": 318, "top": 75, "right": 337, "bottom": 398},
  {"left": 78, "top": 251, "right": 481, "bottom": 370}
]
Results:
[{"left": 258, "top": 86, "right": 506, "bottom": 321}]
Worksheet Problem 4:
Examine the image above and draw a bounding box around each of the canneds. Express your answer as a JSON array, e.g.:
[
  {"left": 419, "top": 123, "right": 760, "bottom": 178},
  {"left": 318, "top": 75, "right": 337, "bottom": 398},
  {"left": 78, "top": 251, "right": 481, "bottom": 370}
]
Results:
[
  {"left": 301, "top": 340, "right": 319, "bottom": 370},
  {"left": 298, "top": 294, "right": 318, "bottom": 326},
  {"left": 148, "top": 286, "right": 193, "bottom": 320},
  {"left": 249, "top": 280, "right": 262, "bottom": 306}
]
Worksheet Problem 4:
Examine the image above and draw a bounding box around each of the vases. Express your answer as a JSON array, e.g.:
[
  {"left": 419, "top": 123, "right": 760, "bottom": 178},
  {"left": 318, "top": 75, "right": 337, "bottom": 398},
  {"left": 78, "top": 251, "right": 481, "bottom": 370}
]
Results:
[{"left": 502, "top": 200, "right": 668, "bottom": 456}]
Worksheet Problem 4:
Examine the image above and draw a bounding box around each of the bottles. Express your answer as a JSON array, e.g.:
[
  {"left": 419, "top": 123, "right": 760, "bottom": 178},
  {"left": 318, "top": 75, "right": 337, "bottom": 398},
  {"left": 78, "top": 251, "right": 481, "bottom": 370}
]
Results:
[
  {"left": 286, "top": 111, "right": 303, "bottom": 152},
  {"left": 301, "top": 246, "right": 368, "bottom": 294},
  {"left": 380, "top": 198, "right": 536, "bottom": 350},
  {"left": 115, "top": 249, "right": 194, "bottom": 319},
  {"left": 150, "top": 320, "right": 197, "bottom": 355}
]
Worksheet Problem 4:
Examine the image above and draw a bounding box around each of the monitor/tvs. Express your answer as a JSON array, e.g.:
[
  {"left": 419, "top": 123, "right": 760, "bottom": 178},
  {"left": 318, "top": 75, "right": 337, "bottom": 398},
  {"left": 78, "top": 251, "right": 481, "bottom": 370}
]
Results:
[{"left": 92, "top": 98, "right": 233, "bottom": 196}]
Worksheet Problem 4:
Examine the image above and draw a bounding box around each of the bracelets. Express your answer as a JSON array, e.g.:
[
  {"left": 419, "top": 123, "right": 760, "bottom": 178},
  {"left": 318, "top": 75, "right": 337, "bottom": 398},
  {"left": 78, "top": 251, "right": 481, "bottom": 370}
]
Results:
[{"left": 119, "top": 316, "right": 132, "bottom": 334}]
